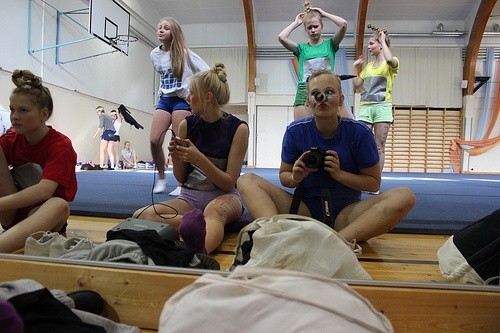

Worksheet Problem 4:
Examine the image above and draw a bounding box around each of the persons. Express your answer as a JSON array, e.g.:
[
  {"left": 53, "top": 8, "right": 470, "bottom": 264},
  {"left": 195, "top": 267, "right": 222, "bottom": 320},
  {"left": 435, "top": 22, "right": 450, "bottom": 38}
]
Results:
[
  {"left": 103, "top": 108, "right": 123, "bottom": 169},
  {"left": 278, "top": 0, "right": 347, "bottom": 121},
  {"left": 149, "top": 17, "right": 210, "bottom": 196},
  {"left": 131, "top": 62, "right": 250, "bottom": 254},
  {"left": 0, "top": 69, "right": 79, "bottom": 253},
  {"left": 237, "top": 69, "right": 416, "bottom": 246},
  {"left": 353, "top": 23, "right": 400, "bottom": 195},
  {"left": 121, "top": 141, "right": 138, "bottom": 168},
  {"left": 91, "top": 106, "right": 116, "bottom": 170}
]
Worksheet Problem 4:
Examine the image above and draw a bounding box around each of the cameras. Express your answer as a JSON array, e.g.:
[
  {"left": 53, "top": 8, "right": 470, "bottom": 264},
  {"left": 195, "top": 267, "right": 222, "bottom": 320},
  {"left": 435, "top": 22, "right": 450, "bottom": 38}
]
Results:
[{"left": 306, "top": 146, "right": 332, "bottom": 171}]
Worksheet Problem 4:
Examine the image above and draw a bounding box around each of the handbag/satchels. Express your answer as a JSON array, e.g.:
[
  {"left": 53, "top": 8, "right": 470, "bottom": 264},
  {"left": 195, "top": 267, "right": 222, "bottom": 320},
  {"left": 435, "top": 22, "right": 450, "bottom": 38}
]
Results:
[{"left": 226, "top": 214, "right": 373, "bottom": 282}]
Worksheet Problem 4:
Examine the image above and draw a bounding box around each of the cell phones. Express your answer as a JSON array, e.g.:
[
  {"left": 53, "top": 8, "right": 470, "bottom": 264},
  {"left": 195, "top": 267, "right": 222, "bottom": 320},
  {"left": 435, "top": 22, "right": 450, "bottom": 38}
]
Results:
[{"left": 175, "top": 137, "right": 189, "bottom": 147}]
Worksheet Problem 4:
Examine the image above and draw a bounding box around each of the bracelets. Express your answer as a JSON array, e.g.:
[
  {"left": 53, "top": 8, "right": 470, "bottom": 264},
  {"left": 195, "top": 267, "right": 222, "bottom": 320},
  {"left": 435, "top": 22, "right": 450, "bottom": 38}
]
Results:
[{"left": 291, "top": 174, "right": 303, "bottom": 183}]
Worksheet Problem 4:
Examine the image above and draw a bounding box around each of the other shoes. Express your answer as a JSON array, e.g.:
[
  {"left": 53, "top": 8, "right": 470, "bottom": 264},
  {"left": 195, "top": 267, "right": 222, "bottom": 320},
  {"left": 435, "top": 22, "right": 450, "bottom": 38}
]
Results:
[
  {"left": 24, "top": 230, "right": 67, "bottom": 258},
  {"left": 186, "top": 251, "right": 220, "bottom": 270},
  {"left": 49, "top": 236, "right": 96, "bottom": 259}
]
